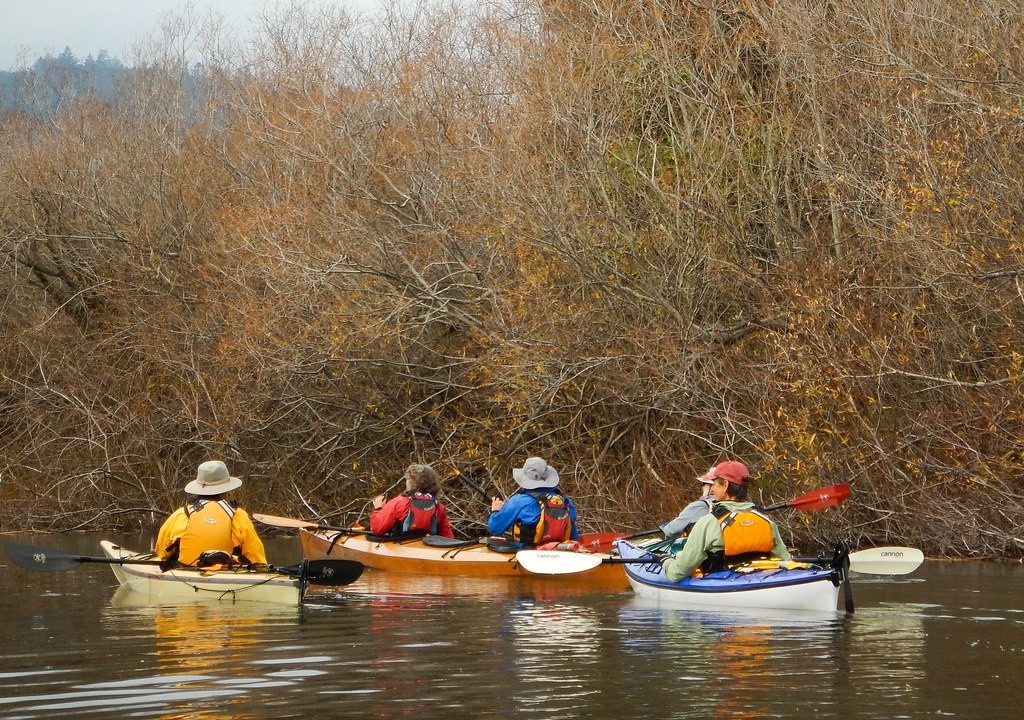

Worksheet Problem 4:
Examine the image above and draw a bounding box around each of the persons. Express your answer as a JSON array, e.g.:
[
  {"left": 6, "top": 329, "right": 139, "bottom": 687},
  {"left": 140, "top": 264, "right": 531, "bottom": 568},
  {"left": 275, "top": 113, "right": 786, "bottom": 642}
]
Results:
[
  {"left": 487, "top": 457, "right": 578, "bottom": 547},
  {"left": 370, "top": 465, "right": 454, "bottom": 539},
  {"left": 652, "top": 461, "right": 794, "bottom": 582},
  {"left": 658, "top": 467, "right": 719, "bottom": 538},
  {"left": 155, "top": 461, "right": 269, "bottom": 572}
]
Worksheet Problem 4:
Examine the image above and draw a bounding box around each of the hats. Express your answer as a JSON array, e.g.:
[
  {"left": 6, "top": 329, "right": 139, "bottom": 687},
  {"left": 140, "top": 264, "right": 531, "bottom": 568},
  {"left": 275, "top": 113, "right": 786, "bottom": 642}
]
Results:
[
  {"left": 696, "top": 460, "right": 755, "bottom": 489},
  {"left": 184, "top": 460, "right": 242, "bottom": 496},
  {"left": 513, "top": 456, "right": 560, "bottom": 490}
]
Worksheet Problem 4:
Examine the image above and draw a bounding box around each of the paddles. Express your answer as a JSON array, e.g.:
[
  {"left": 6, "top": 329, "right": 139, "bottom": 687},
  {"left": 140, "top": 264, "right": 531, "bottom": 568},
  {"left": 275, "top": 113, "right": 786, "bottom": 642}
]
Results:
[
  {"left": 422, "top": 536, "right": 532, "bottom": 554},
  {"left": 7, "top": 542, "right": 366, "bottom": 586},
  {"left": 579, "top": 484, "right": 851, "bottom": 551},
  {"left": 428, "top": 451, "right": 522, "bottom": 529},
  {"left": 516, "top": 548, "right": 925, "bottom": 575},
  {"left": 253, "top": 513, "right": 390, "bottom": 540}
]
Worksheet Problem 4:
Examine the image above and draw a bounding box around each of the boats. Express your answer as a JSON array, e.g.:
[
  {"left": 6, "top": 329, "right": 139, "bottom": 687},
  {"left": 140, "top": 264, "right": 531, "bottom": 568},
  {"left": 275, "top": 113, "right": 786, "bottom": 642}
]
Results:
[
  {"left": 100, "top": 540, "right": 309, "bottom": 605},
  {"left": 611, "top": 538, "right": 798, "bottom": 557},
  {"left": 298, "top": 526, "right": 631, "bottom": 590},
  {"left": 615, "top": 537, "right": 849, "bottom": 612}
]
